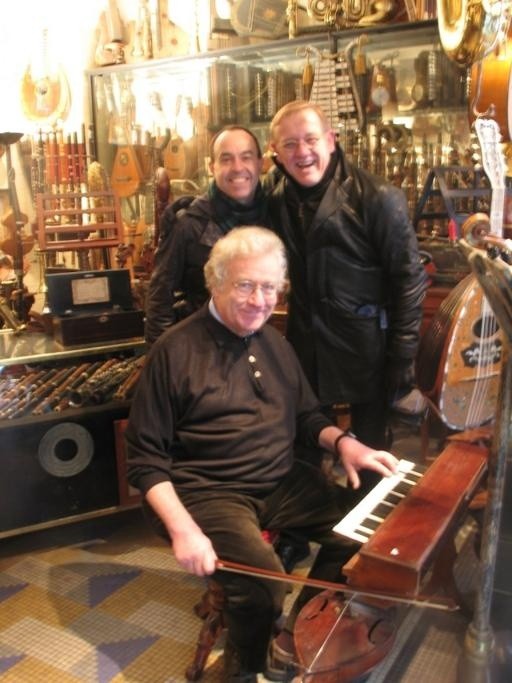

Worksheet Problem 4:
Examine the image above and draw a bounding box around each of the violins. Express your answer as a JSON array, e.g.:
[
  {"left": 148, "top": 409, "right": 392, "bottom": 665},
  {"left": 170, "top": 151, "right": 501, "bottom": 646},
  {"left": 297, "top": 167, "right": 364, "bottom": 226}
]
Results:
[{"left": 2, "top": 168, "right": 33, "bottom": 256}]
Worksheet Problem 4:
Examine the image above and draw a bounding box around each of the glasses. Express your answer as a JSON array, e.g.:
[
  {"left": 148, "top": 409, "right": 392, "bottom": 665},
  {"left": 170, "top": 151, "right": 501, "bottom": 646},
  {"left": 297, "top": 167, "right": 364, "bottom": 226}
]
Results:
[
  {"left": 277, "top": 130, "right": 329, "bottom": 151},
  {"left": 226, "top": 276, "right": 280, "bottom": 295}
]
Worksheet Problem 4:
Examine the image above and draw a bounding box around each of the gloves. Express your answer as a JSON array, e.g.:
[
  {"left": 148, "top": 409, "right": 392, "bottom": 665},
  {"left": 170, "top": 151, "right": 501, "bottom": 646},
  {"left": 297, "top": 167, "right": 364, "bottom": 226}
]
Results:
[{"left": 384, "top": 362, "right": 417, "bottom": 392}]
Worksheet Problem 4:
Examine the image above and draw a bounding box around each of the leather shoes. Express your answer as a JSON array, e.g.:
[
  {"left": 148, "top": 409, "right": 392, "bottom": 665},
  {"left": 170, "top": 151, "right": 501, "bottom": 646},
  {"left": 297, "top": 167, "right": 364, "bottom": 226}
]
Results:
[
  {"left": 263, "top": 638, "right": 299, "bottom": 682},
  {"left": 220, "top": 637, "right": 258, "bottom": 683}
]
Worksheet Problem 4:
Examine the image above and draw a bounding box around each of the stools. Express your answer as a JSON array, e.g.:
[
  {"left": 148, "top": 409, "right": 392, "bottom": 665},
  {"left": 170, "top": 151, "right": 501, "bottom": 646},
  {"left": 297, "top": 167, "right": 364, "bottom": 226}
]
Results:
[{"left": 184, "top": 529, "right": 293, "bottom": 682}]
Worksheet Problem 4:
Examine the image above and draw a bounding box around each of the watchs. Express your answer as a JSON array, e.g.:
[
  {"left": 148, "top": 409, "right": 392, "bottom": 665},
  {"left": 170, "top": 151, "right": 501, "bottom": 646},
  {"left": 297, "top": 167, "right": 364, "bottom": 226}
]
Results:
[{"left": 333, "top": 426, "right": 357, "bottom": 458}]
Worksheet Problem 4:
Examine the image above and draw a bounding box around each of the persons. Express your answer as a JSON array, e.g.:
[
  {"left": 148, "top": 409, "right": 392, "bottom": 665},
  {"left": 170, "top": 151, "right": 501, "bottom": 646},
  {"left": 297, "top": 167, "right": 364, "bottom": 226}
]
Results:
[
  {"left": 140, "top": 122, "right": 279, "bottom": 353},
  {"left": 155, "top": 97, "right": 429, "bottom": 578},
  {"left": 124, "top": 223, "right": 404, "bottom": 682}
]
